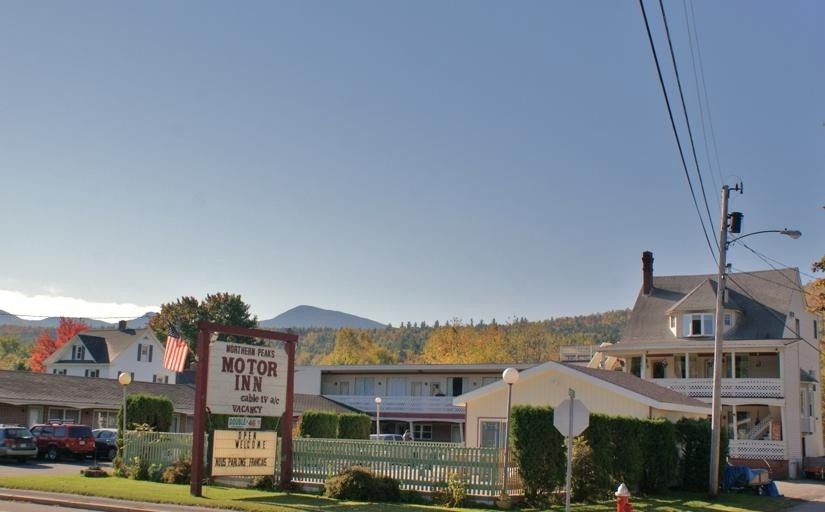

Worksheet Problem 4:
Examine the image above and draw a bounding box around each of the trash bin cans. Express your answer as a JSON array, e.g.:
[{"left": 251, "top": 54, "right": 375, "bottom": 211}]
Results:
[{"left": 788, "top": 460, "right": 797, "bottom": 480}]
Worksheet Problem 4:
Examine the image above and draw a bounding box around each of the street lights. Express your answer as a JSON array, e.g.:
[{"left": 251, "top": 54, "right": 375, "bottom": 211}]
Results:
[
  {"left": 375, "top": 397, "right": 382, "bottom": 442},
  {"left": 710, "top": 229, "right": 801, "bottom": 495},
  {"left": 119, "top": 372, "right": 132, "bottom": 465},
  {"left": 501, "top": 367, "right": 520, "bottom": 494}
]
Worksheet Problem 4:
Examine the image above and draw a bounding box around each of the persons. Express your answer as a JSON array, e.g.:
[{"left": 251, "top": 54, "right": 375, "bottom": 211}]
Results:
[
  {"left": 402, "top": 430, "right": 410, "bottom": 441},
  {"left": 431, "top": 384, "right": 441, "bottom": 396}
]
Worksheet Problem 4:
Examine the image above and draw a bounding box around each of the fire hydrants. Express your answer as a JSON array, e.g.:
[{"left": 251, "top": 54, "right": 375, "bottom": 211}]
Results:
[{"left": 614, "top": 482, "right": 633, "bottom": 512}]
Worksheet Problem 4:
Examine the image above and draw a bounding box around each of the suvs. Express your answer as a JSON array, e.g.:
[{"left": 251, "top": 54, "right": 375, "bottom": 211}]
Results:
[{"left": 29, "top": 419, "right": 96, "bottom": 461}]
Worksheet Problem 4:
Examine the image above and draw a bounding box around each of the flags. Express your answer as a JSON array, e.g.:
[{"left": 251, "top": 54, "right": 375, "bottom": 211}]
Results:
[{"left": 162, "top": 325, "right": 188, "bottom": 372}]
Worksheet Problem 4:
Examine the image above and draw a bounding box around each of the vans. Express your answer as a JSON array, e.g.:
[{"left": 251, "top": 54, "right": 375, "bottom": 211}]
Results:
[{"left": 369, "top": 434, "right": 403, "bottom": 441}]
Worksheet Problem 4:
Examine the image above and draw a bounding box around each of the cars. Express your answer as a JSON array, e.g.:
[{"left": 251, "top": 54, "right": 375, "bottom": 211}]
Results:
[
  {"left": 92, "top": 429, "right": 119, "bottom": 462},
  {"left": 0, "top": 423, "right": 39, "bottom": 463}
]
